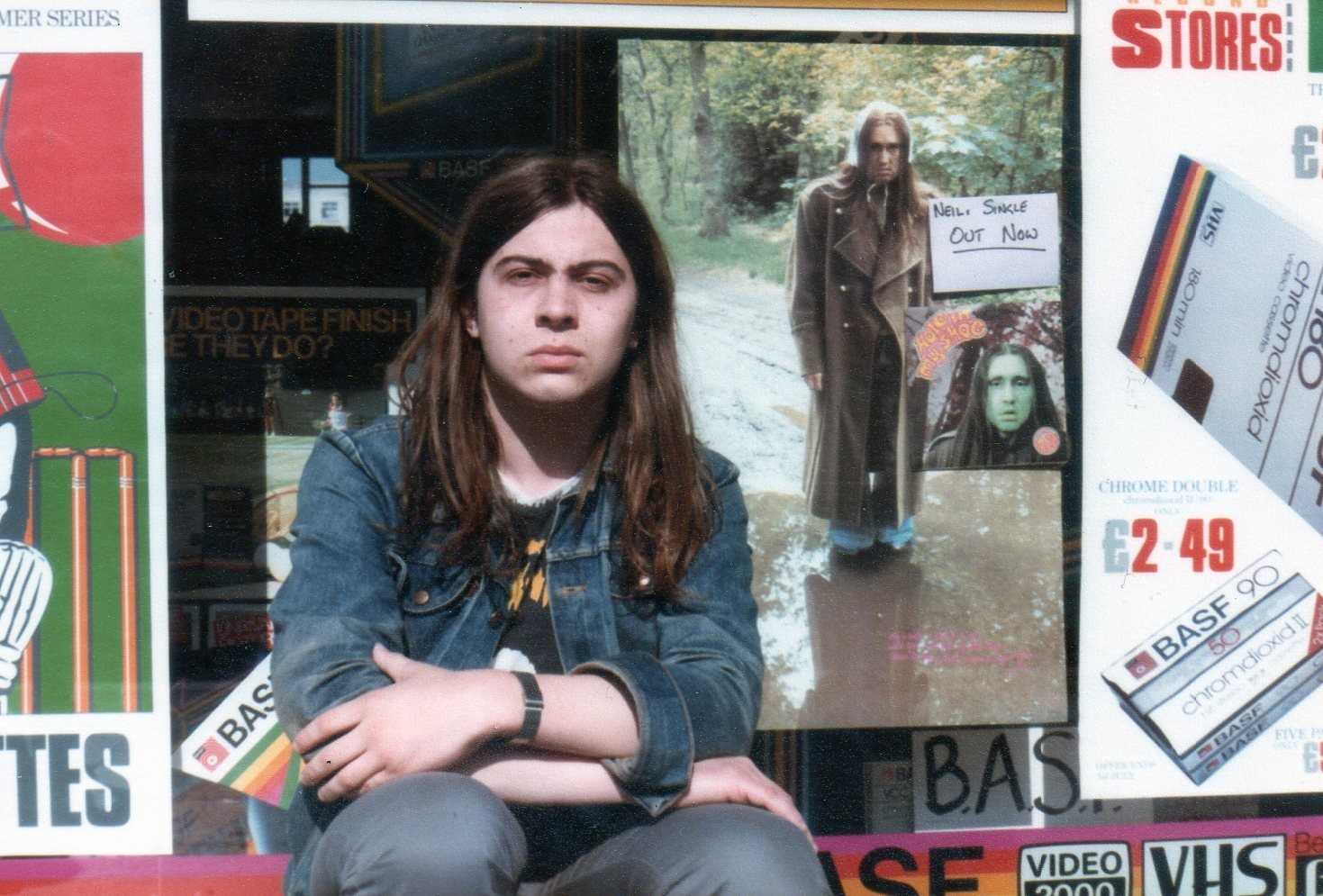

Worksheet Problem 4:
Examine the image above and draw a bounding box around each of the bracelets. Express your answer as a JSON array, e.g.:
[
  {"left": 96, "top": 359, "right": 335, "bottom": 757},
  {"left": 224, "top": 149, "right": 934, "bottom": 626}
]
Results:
[{"left": 508, "top": 671, "right": 543, "bottom": 748}]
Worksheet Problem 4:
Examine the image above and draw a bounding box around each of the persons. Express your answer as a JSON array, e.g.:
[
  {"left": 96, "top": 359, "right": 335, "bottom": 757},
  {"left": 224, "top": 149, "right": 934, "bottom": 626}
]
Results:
[
  {"left": 327, "top": 392, "right": 351, "bottom": 431},
  {"left": 804, "top": 550, "right": 930, "bottom": 729},
  {"left": 785, "top": 104, "right": 939, "bottom": 561},
  {"left": 924, "top": 344, "right": 1066, "bottom": 469},
  {"left": 268, "top": 157, "right": 830, "bottom": 896}
]
